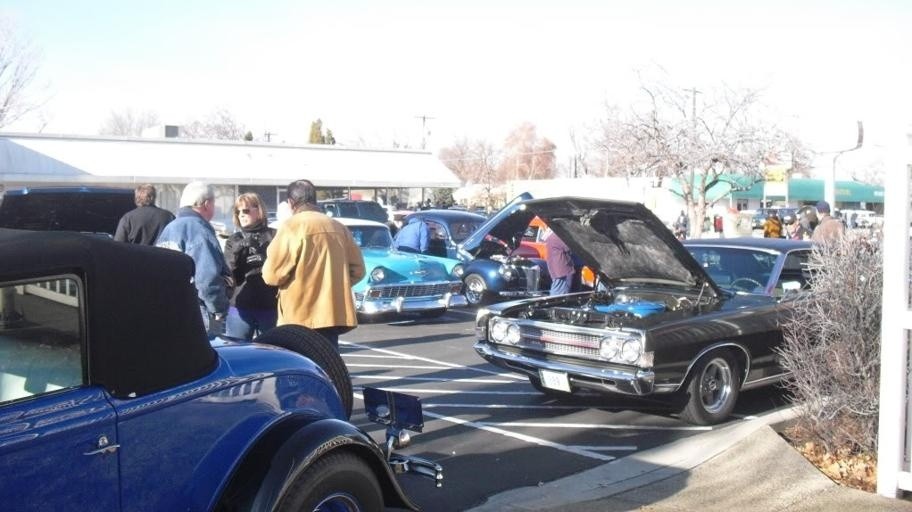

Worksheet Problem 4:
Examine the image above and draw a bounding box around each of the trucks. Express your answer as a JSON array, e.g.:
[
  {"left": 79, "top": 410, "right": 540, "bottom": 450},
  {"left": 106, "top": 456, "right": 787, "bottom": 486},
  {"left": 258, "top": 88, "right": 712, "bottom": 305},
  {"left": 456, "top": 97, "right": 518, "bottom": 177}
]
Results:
[{"left": 847, "top": 210, "right": 883, "bottom": 228}]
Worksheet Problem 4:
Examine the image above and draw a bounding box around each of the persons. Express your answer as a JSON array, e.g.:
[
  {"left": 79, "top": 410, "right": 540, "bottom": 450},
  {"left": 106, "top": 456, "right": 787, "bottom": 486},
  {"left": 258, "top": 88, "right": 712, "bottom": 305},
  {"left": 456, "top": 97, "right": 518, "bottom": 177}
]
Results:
[
  {"left": 259, "top": 179, "right": 365, "bottom": 352},
  {"left": 674, "top": 210, "right": 689, "bottom": 241},
  {"left": 221, "top": 191, "right": 279, "bottom": 342},
  {"left": 112, "top": 183, "right": 175, "bottom": 248},
  {"left": 393, "top": 218, "right": 430, "bottom": 255},
  {"left": 154, "top": 181, "right": 231, "bottom": 338},
  {"left": 761, "top": 199, "right": 847, "bottom": 247}
]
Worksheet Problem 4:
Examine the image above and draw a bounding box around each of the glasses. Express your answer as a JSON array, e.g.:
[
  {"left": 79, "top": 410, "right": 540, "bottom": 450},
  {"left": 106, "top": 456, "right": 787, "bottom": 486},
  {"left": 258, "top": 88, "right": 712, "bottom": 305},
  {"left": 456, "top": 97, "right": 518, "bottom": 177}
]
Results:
[{"left": 235, "top": 207, "right": 258, "bottom": 216}]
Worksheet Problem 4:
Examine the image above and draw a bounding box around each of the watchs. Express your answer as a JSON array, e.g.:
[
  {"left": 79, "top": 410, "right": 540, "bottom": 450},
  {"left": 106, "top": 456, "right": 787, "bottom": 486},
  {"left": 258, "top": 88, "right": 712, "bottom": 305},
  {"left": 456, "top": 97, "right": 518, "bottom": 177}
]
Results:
[{"left": 544, "top": 231, "right": 576, "bottom": 295}]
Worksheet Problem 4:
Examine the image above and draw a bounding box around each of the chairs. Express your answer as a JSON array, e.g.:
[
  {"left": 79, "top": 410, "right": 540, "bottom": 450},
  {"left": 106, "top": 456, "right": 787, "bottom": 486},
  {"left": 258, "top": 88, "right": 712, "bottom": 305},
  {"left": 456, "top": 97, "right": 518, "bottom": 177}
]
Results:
[{"left": 732, "top": 251, "right": 811, "bottom": 291}]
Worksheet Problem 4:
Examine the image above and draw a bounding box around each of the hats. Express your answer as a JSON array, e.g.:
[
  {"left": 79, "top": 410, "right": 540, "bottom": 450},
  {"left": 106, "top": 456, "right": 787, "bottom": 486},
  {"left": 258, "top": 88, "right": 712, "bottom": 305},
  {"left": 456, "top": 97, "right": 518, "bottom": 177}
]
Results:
[
  {"left": 180, "top": 181, "right": 214, "bottom": 208},
  {"left": 782, "top": 213, "right": 797, "bottom": 225},
  {"left": 814, "top": 200, "right": 831, "bottom": 215}
]
